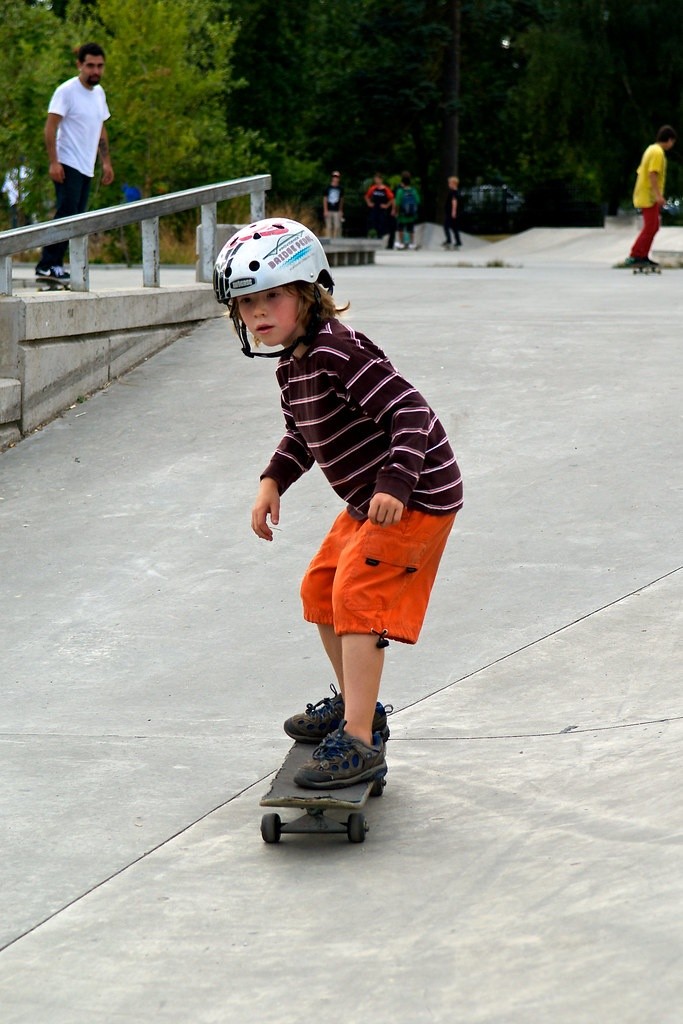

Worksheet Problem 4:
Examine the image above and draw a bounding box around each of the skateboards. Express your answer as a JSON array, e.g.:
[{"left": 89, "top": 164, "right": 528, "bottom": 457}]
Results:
[
  {"left": 631, "top": 263, "right": 662, "bottom": 275},
  {"left": 35, "top": 277, "right": 71, "bottom": 291},
  {"left": 442, "top": 245, "right": 459, "bottom": 251},
  {"left": 259, "top": 739, "right": 388, "bottom": 844}
]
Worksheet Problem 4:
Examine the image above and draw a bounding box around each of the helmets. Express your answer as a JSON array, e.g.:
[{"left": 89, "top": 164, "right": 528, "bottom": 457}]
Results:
[{"left": 212, "top": 217, "right": 336, "bottom": 305}]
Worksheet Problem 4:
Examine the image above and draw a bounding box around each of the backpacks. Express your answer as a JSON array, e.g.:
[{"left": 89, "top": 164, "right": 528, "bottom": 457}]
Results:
[{"left": 398, "top": 188, "right": 418, "bottom": 217}]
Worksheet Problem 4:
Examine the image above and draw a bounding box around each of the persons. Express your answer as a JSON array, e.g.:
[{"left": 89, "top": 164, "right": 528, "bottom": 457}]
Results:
[
  {"left": 365, "top": 174, "right": 394, "bottom": 239},
  {"left": 210, "top": 217, "right": 463, "bottom": 790},
  {"left": 322, "top": 170, "right": 344, "bottom": 238},
  {"left": 34, "top": 42, "right": 115, "bottom": 283},
  {"left": 390, "top": 175, "right": 422, "bottom": 250},
  {"left": 621, "top": 126, "right": 677, "bottom": 267},
  {"left": 443, "top": 176, "right": 462, "bottom": 247}
]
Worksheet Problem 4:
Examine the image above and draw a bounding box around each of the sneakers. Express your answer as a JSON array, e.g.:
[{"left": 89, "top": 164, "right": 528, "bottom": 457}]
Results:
[
  {"left": 36, "top": 265, "right": 70, "bottom": 281},
  {"left": 293, "top": 719, "right": 388, "bottom": 790},
  {"left": 283, "top": 684, "right": 394, "bottom": 742}
]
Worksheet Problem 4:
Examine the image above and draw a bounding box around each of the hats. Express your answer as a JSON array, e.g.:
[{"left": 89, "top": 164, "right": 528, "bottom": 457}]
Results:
[{"left": 332, "top": 171, "right": 341, "bottom": 176}]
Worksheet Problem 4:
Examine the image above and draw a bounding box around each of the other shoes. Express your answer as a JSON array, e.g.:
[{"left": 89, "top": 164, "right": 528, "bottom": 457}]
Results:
[{"left": 626, "top": 254, "right": 657, "bottom": 266}]
